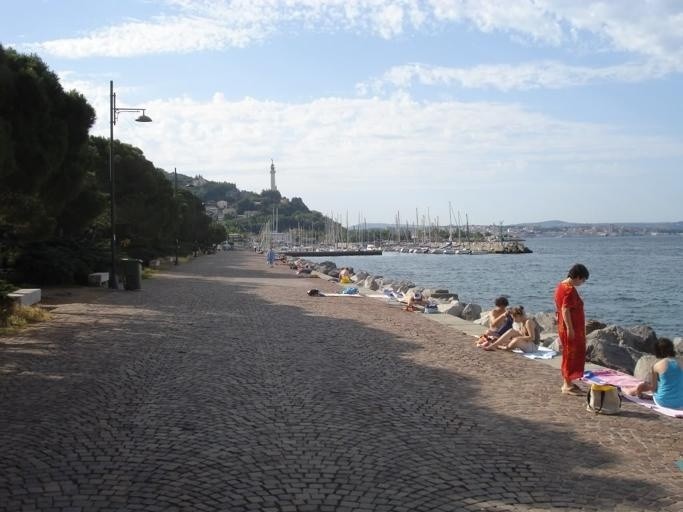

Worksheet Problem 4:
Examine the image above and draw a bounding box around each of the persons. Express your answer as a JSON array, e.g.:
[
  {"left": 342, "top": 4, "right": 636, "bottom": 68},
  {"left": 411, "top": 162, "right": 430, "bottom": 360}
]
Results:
[
  {"left": 338, "top": 267, "right": 349, "bottom": 282},
  {"left": 476, "top": 297, "right": 540, "bottom": 353},
  {"left": 629, "top": 338, "right": 682, "bottom": 409},
  {"left": 267, "top": 248, "right": 308, "bottom": 273},
  {"left": 554, "top": 263, "right": 589, "bottom": 396}
]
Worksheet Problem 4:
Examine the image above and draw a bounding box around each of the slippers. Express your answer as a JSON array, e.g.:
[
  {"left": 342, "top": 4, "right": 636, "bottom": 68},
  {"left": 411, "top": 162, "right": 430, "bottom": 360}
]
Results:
[{"left": 561, "top": 385, "right": 582, "bottom": 396}]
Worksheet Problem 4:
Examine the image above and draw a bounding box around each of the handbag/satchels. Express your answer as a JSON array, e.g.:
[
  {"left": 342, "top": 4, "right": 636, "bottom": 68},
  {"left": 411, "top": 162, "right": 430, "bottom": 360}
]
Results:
[{"left": 586, "top": 384, "right": 622, "bottom": 414}]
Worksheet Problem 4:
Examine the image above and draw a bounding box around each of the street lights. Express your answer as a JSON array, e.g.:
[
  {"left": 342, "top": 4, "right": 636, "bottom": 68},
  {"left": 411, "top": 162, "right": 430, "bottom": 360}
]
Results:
[
  {"left": 173, "top": 168, "right": 194, "bottom": 265},
  {"left": 108, "top": 80, "right": 152, "bottom": 291}
]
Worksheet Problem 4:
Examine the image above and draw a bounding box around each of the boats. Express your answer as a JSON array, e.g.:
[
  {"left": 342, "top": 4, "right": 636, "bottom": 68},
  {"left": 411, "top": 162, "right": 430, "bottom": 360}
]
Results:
[{"left": 255, "top": 201, "right": 533, "bottom": 257}]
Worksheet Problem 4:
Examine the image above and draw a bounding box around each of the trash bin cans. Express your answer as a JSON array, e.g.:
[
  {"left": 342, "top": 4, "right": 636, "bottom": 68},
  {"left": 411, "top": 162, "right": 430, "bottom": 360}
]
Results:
[{"left": 121, "top": 258, "right": 144, "bottom": 290}]
